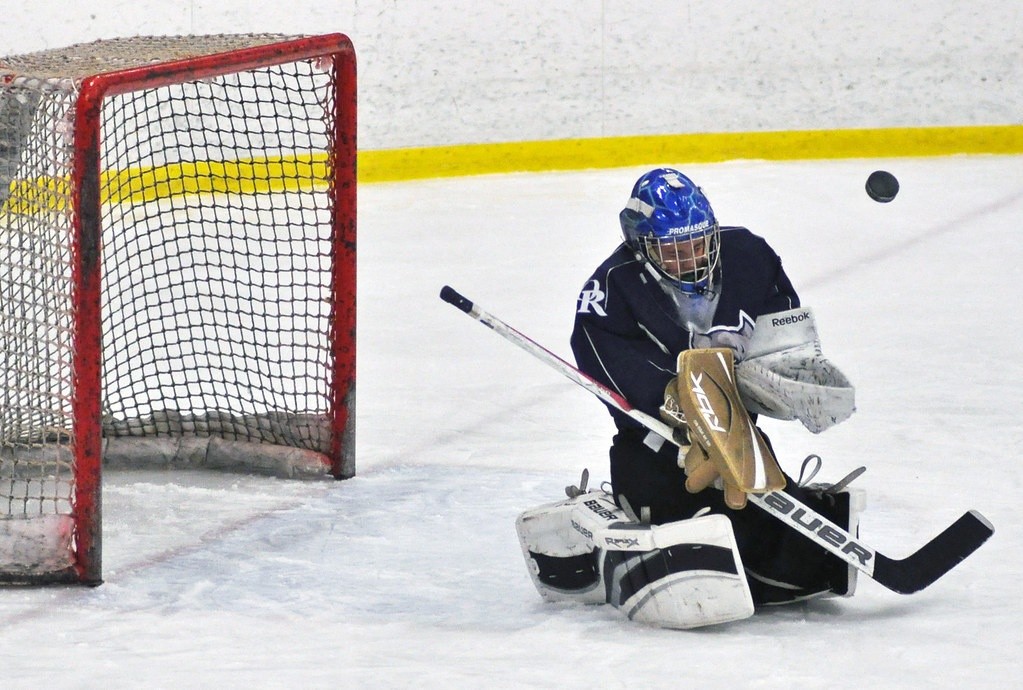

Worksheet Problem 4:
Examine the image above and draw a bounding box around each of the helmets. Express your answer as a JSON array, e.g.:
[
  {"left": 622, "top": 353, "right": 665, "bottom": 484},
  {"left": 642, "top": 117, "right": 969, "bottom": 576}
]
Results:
[{"left": 619, "top": 167, "right": 723, "bottom": 302}]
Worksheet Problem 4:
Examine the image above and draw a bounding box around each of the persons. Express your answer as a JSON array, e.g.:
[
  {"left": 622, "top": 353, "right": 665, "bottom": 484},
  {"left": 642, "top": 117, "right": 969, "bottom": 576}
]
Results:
[{"left": 515, "top": 169, "right": 858, "bottom": 627}]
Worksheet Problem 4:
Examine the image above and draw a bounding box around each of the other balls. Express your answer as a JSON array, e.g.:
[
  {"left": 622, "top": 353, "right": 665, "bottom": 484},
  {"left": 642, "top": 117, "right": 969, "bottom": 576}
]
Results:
[{"left": 865, "top": 169, "right": 900, "bottom": 204}]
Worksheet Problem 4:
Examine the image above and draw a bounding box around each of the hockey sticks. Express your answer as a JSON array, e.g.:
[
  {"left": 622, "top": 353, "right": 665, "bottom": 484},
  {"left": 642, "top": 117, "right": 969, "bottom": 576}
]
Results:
[{"left": 438, "top": 286, "right": 997, "bottom": 595}]
